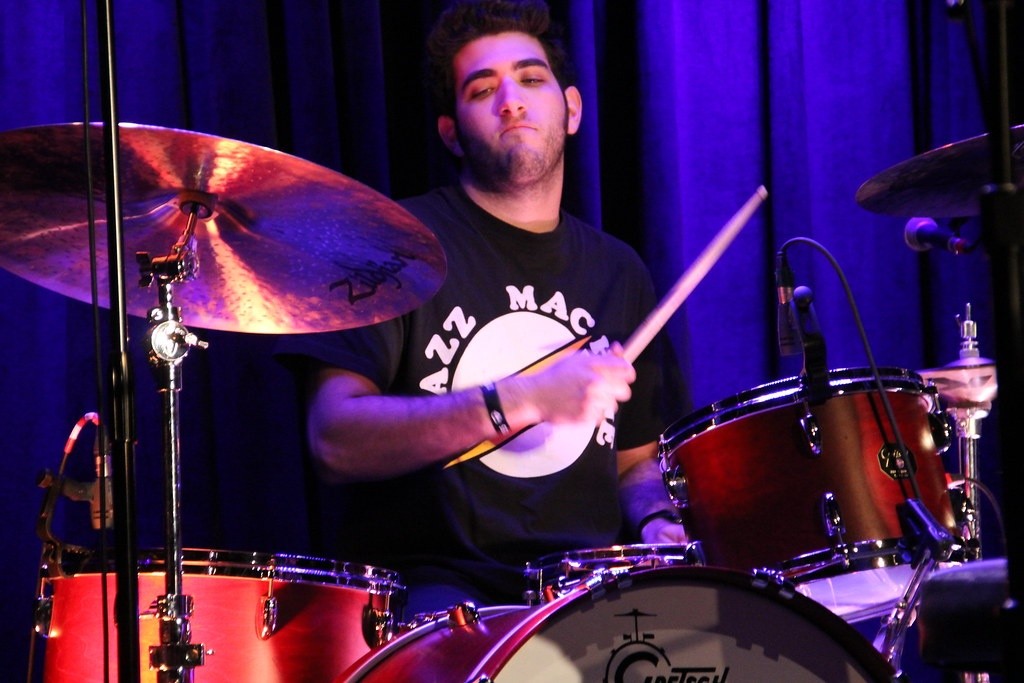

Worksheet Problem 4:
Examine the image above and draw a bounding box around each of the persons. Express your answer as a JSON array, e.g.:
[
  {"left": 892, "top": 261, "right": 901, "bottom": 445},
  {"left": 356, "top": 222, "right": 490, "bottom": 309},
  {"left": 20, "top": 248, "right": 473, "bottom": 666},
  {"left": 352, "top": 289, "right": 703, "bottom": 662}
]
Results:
[{"left": 271, "top": 12, "right": 693, "bottom": 624}]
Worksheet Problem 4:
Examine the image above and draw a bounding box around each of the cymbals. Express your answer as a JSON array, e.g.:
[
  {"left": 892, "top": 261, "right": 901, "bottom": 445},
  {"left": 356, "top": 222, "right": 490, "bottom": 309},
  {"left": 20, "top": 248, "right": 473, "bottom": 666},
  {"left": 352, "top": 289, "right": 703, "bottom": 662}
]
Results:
[
  {"left": 854, "top": 122, "right": 1024, "bottom": 218},
  {"left": 0, "top": 123, "right": 449, "bottom": 336}
]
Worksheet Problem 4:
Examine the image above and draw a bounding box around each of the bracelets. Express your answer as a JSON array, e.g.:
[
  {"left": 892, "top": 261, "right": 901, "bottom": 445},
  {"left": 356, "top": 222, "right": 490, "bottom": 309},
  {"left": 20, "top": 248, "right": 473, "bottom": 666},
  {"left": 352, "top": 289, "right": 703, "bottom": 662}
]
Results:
[
  {"left": 637, "top": 508, "right": 683, "bottom": 535},
  {"left": 480, "top": 383, "right": 511, "bottom": 438}
]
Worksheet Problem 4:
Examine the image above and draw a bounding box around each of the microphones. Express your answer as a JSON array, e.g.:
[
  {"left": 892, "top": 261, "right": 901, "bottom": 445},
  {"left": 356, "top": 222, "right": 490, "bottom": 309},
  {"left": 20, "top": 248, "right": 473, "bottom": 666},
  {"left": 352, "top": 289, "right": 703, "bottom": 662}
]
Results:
[
  {"left": 91, "top": 427, "right": 114, "bottom": 530},
  {"left": 905, "top": 217, "right": 968, "bottom": 255},
  {"left": 774, "top": 251, "right": 804, "bottom": 357}
]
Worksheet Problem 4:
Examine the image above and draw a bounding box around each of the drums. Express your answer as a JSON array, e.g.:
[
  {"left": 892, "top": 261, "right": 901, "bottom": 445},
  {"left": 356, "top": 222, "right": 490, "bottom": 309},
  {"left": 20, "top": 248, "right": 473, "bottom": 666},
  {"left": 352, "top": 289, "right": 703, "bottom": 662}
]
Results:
[
  {"left": 519, "top": 541, "right": 695, "bottom": 606},
  {"left": 330, "top": 568, "right": 906, "bottom": 683},
  {"left": 28, "top": 545, "right": 409, "bottom": 683},
  {"left": 658, "top": 363, "right": 971, "bottom": 626}
]
